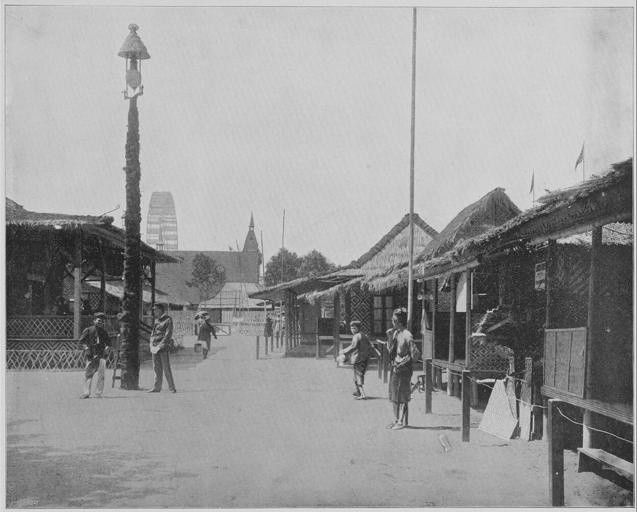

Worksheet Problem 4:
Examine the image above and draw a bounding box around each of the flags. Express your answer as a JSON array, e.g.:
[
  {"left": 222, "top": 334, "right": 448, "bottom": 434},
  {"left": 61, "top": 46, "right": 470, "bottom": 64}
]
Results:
[
  {"left": 529, "top": 173, "right": 535, "bottom": 193},
  {"left": 574, "top": 145, "right": 583, "bottom": 172}
]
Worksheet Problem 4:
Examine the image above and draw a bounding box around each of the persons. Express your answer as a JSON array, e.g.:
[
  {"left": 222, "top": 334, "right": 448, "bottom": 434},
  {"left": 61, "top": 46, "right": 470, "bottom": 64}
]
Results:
[
  {"left": 147, "top": 302, "right": 177, "bottom": 394},
  {"left": 340, "top": 319, "right": 371, "bottom": 399},
  {"left": 196, "top": 317, "right": 216, "bottom": 359},
  {"left": 384, "top": 309, "right": 419, "bottom": 429},
  {"left": 78, "top": 311, "right": 113, "bottom": 400}
]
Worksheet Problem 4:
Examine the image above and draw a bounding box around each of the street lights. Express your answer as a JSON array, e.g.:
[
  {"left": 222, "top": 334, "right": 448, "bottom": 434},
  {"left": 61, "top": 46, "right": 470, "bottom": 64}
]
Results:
[{"left": 115, "top": 21, "right": 151, "bottom": 391}]
